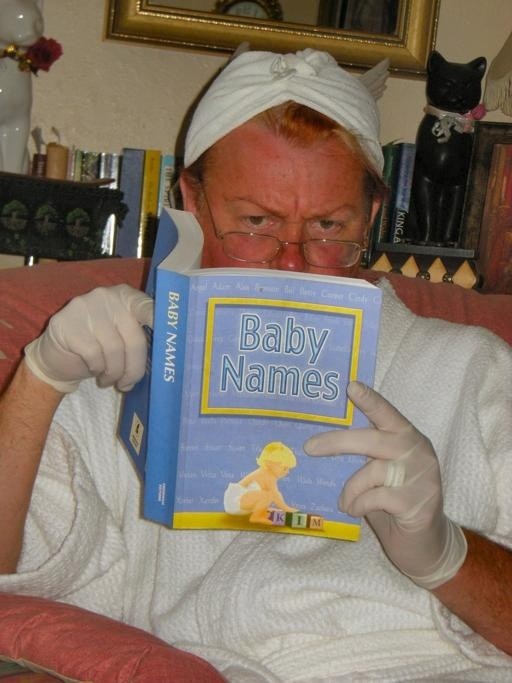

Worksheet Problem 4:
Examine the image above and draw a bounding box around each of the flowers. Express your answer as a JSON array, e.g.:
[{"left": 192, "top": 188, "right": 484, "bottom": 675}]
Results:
[{"left": 18, "top": 36, "right": 63, "bottom": 79}]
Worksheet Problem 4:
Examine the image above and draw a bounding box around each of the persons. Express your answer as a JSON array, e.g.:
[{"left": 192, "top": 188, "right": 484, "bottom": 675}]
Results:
[
  {"left": 224, "top": 441, "right": 300, "bottom": 524},
  {"left": 0, "top": 47, "right": 512, "bottom": 683}
]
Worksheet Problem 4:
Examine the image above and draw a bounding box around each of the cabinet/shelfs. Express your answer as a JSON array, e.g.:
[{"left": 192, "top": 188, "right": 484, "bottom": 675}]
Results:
[{"left": 462, "top": 118, "right": 512, "bottom": 296}]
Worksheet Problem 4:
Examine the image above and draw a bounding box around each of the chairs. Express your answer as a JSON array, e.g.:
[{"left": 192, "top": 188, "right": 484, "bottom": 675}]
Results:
[{"left": 3, "top": 258, "right": 512, "bottom": 683}]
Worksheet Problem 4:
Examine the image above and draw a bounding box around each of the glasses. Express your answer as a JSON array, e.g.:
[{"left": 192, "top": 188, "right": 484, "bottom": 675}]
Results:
[{"left": 192, "top": 164, "right": 371, "bottom": 270}]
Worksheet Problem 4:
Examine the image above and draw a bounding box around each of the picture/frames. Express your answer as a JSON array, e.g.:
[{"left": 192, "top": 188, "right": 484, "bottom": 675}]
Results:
[{"left": 100, "top": 0, "right": 444, "bottom": 82}]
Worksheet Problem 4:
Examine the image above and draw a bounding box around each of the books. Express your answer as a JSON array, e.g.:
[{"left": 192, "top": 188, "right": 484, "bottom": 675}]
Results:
[{"left": 115, "top": 207, "right": 383, "bottom": 541}]
